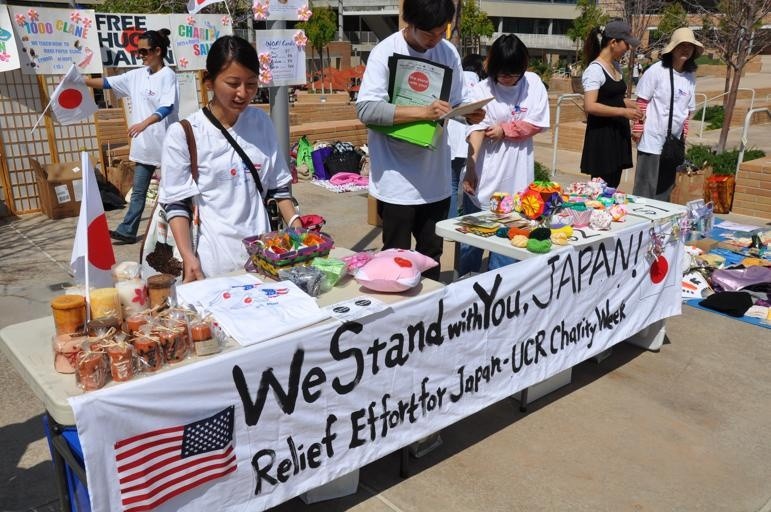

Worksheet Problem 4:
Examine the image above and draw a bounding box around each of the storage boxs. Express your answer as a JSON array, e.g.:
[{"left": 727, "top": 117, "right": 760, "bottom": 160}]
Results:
[
  {"left": 27, "top": 156, "right": 99, "bottom": 220},
  {"left": 297, "top": 469, "right": 359, "bottom": 505},
  {"left": 513, "top": 369, "right": 572, "bottom": 405},
  {"left": 367, "top": 195, "right": 383, "bottom": 228}
]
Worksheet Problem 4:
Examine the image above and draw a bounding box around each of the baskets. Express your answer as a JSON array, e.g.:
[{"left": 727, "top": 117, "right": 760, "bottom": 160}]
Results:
[{"left": 243, "top": 225, "right": 334, "bottom": 280}]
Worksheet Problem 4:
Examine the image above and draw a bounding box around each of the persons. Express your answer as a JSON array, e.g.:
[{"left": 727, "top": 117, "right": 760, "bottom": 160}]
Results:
[
  {"left": 616, "top": 46, "right": 653, "bottom": 86},
  {"left": 580, "top": 22, "right": 646, "bottom": 188},
  {"left": 80, "top": 28, "right": 180, "bottom": 244},
  {"left": 633, "top": 28, "right": 704, "bottom": 201},
  {"left": 356, "top": 1, "right": 486, "bottom": 281},
  {"left": 453, "top": 34, "right": 550, "bottom": 280},
  {"left": 566, "top": 60, "right": 584, "bottom": 77},
  {"left": 443, "top": 54, "right": 488, "bottom": 241},
  {"left": 156, "top": 36, "right": 303, "bottom": 285}
]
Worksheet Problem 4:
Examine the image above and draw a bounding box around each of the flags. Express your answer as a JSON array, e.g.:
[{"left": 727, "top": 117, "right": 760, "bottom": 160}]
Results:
[
  {"left": 70, "top": 150, "right": 116, "bottom": 289},
  {"left": 187, "top": 0, "right": 221, "bottom": 15},
  {"left": 50, "top": 63, "right": 98, "bottom": 126}
]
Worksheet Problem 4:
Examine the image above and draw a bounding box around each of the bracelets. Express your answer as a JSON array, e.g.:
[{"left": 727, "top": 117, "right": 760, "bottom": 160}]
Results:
[{"left": 287, "top": 215, "right": 299, "bottom": 228}]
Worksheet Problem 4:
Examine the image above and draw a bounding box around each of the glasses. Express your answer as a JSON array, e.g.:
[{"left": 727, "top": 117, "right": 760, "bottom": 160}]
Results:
[
  {"left": 138, "top": 48, "right": 152, "bottom": 56},
  {"left": 495, "top": 76, "right": 522, "bottom": 79}
]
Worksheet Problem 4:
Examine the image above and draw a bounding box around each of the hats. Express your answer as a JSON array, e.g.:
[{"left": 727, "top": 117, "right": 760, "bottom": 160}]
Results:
[
  {"left": 661, "top": 28, "right": 704, "bottom": 59},
  {"left": 605, "top": 21, "right": 642, "bottom": 47}
]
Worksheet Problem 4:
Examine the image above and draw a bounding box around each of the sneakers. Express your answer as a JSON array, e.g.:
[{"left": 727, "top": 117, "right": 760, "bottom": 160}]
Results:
[{"left": 108, "top": 229, "right": 137, "bottom": 244}]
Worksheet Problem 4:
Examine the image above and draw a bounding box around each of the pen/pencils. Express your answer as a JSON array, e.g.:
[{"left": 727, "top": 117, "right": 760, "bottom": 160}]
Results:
[{"left": 432, "top": 94, "right": 439, "bottom": 100}]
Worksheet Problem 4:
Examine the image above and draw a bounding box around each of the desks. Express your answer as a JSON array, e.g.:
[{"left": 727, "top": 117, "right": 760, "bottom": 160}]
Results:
[
  {"left": 434, "top": 188, "right": 690, "bottom": 404},
  {"left": 0, "top": 245, "right": 446, "bottom": 512}
]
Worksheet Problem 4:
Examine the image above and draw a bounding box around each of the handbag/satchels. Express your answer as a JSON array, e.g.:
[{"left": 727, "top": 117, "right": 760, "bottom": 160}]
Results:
[
  {"left": 692, "top": 202, "right": 714, "bottom": 236},
  {"left": 710, "top": 263, "right": 767, "bottom": 294},
  {"left": 140, "top": 195, "right": 199, "bottom": 285},
  {"left": 289, "top": 133, "right": 370, "bottom": 181},
  {"left": 661, "top": 133, "right": 685, "bottom": 167},
  {"left": 98, "top": 161, "right": 160, "bottom": 211}
]
